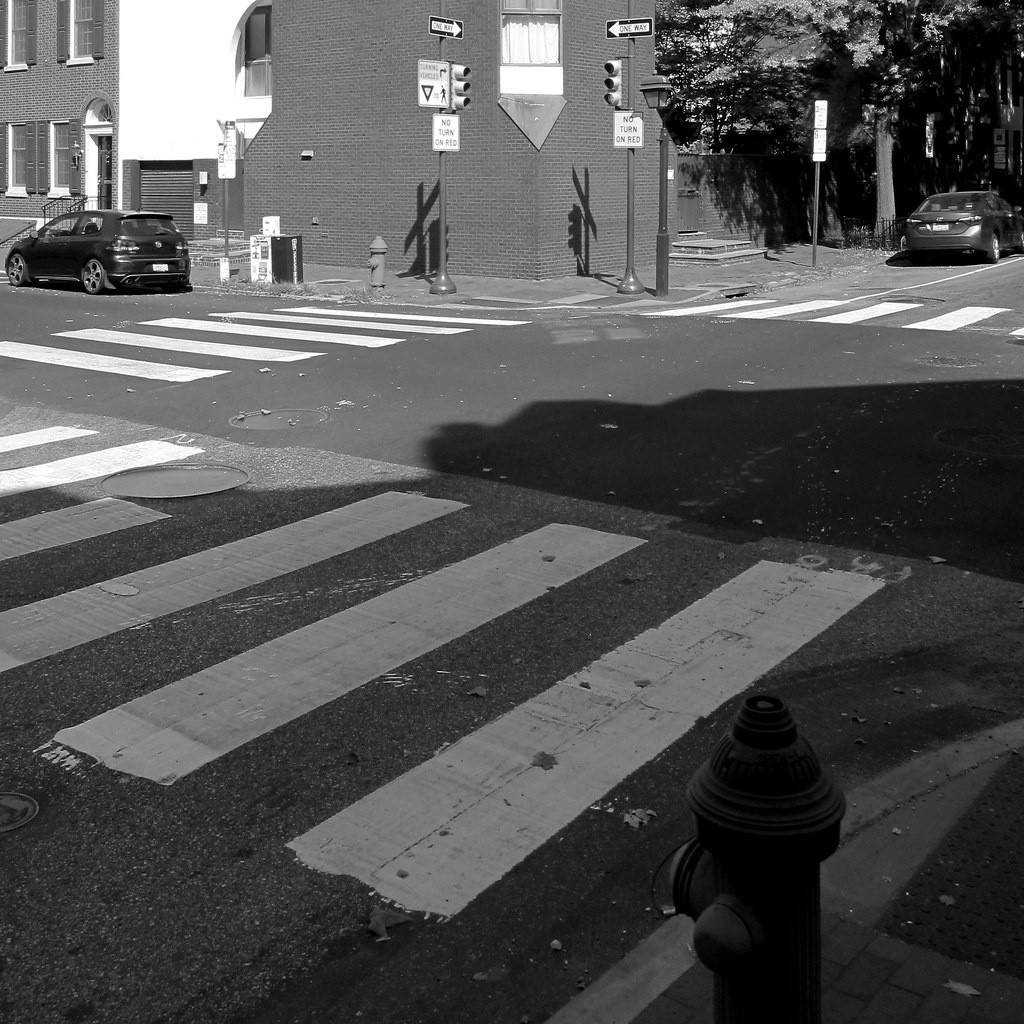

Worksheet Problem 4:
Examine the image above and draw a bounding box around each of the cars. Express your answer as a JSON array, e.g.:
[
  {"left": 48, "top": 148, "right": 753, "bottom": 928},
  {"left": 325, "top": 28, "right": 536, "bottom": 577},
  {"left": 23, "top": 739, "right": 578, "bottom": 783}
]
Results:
[
  {"left": 904, "top": 191, "right": 1022, "bottom": 264},
  {"left": 6, "top": 210, "right": 190, "bottom": 297}
]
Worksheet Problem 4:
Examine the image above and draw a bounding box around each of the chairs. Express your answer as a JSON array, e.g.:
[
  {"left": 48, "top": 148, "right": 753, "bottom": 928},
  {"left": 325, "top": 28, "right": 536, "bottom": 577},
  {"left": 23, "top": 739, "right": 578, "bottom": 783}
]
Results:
[{"left": 85, "top": 223, "right": 99, "bottom": 235}]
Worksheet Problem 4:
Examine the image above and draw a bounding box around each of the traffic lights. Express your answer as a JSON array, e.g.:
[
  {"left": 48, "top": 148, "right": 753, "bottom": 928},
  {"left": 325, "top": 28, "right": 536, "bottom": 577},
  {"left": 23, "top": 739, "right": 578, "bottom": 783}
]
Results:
[
  {"left": 450, "top": 62, "right": 470, "bottom": 110},
  {"left": 603, "top": 59, "right": 623, "bottom": 106}
]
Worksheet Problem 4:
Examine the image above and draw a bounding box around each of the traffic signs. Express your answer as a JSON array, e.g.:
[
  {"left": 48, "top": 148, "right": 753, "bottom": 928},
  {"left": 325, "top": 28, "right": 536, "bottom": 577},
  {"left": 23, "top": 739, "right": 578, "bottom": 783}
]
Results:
[
  {"left": 605, "top": 17, "right": 655, "bottom": 39},
  {"left": 428, "top": 14, "right": 464, "bottom": 39}
]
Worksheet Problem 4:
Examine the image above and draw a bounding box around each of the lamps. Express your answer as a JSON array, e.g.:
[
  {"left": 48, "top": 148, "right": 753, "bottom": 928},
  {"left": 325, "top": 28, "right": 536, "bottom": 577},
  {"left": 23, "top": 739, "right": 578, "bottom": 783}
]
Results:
[
  {"left": 70, "top": 140, "right": 84, "bottom": 167},
  {"left": 301, "top": 150, "right": 313, "bottom": 158}
]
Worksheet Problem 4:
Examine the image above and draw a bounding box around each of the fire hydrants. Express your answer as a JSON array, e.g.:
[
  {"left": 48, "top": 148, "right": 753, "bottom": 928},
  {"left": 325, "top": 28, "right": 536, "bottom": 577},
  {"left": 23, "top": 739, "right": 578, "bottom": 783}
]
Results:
[
  {"left": 366, "top": 236, "right": 388, "bottom": 293},
  {"left": 668, "top": 691, "right": 844, "bottom": 1024}
]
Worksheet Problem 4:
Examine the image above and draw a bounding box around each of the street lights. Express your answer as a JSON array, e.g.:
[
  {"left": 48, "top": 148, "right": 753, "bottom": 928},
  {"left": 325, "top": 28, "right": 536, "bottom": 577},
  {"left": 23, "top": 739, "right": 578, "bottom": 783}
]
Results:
[{"left": 639, "top": 73, "right": 677, "bottom": 297}]
